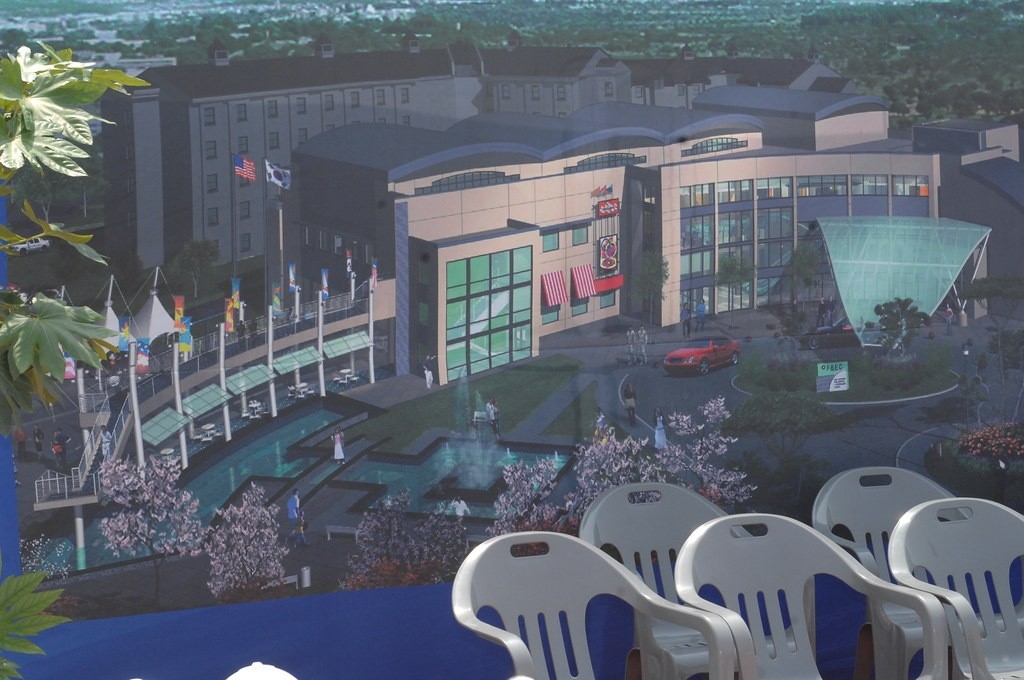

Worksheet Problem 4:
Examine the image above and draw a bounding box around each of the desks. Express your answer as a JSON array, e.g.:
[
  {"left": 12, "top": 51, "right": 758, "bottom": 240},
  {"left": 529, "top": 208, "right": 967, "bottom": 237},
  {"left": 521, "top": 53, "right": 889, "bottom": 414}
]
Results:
[
  {"left": 249, "top": 403, "right": 260, "bottom": 416},
  {"left": 295, "top": 383, "right": 307, "bottom": 395},
  {"left": 339, "top": 368, "right": 351, "bottom": 380},
  {"left": 160, "top": 448, "right": 174, "bottom": 461},
  {"left": 201, "top": 423, "right": 215, "bottom": 437}
]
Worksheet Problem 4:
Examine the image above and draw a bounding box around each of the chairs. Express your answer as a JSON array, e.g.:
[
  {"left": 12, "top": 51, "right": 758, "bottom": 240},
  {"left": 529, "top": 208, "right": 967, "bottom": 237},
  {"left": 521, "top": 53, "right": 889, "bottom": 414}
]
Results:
[
  {"left": 333, "top": 372, "right": 360, "bottom": 387},
  {"left": 192, "top": 428, "right": 224, "bottom": 442},
  {"left": 289, "top": 387, "right": 315, "bottom": 399},
  {"left": 452, "top": 462, "right": 1024, "bottom": 680},
  {"left": 241, "top": 407, "right": 269, "bottom": 420}
]
